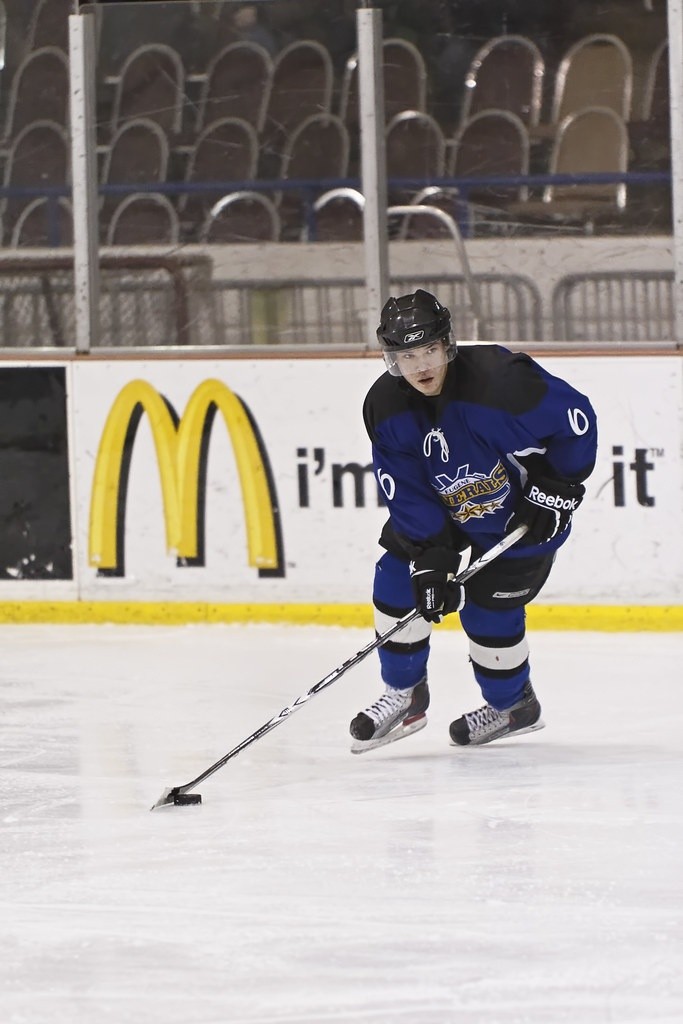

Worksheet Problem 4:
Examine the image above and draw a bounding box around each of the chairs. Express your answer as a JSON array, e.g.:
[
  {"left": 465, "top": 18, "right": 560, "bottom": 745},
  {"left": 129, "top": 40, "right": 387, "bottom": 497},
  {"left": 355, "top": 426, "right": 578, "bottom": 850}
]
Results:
[{"left": 0, "top": 0, "right": 683, "bottom": 248}]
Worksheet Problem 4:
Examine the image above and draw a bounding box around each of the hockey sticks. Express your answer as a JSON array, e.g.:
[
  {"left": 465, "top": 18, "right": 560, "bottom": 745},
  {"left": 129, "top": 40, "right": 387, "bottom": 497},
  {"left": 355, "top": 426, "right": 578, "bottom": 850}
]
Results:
[{"left": 149, "top": 523, "right": 530, "bottom": 812}]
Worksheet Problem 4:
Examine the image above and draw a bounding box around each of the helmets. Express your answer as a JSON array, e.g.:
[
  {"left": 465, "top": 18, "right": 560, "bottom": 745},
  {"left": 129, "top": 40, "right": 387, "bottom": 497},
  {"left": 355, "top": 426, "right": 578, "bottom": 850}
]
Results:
[{"left": 376, "top": 289, "right": 458, "bottom": 376}]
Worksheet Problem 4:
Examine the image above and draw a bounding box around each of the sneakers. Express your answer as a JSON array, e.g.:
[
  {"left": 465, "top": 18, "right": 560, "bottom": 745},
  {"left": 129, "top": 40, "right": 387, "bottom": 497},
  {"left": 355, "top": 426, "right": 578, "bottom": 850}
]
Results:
[
  {"left": 350, "top": 669, "right": 430, "bottom": 755},
  {"left": 450, "top": 680, "right": 545, "bottom": 747}
]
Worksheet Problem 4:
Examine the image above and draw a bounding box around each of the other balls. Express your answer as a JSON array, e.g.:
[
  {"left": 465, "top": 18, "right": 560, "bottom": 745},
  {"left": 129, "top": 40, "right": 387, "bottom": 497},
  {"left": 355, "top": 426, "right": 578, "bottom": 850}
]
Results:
[{"left": 173, "top": 793, "right": 202, "bottom": 807}]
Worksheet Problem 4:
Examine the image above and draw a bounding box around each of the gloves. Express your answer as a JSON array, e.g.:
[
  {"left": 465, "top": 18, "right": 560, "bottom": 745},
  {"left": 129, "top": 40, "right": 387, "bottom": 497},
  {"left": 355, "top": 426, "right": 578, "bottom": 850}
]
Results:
[
  {"left": 504, "top": 469, "right": 585, "bottom": 548},
  {"left": 408, "top": 545, "right": 472, "bottom": 624}
]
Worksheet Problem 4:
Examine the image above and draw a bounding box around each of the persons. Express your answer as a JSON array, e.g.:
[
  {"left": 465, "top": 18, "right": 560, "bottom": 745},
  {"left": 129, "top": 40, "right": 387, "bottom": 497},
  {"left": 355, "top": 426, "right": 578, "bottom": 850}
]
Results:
[{"left": 349, "top": 289, "right": 597, "bottom": 747}]
